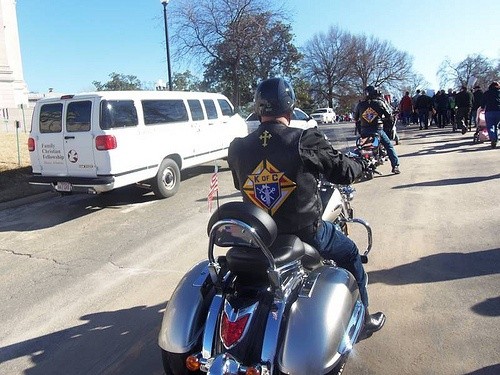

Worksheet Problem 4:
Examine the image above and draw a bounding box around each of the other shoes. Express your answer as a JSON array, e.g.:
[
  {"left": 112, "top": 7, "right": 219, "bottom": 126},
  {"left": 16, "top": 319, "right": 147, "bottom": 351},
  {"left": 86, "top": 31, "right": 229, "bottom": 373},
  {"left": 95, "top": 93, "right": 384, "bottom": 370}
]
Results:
[
  {"left": 392, "top": 166, "right": 400, "bottom": 174},
  {"left": 462, "top": 127, "right": 467, "bottom": 134},
  {"left": 491, "top": 142, "right": 496, "bottom": 148}
]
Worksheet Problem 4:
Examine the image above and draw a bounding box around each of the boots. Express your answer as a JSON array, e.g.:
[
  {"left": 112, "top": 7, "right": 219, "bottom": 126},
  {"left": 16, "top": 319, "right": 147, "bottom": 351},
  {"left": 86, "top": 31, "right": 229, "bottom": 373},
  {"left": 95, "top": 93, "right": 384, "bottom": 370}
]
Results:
[{"left": 361, "top": 308, "right": 386, "bottom": 337}]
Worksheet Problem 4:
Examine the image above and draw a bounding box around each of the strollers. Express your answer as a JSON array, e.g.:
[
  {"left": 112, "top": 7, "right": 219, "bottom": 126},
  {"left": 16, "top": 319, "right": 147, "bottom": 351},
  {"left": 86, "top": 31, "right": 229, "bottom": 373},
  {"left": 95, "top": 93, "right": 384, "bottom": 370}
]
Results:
[{"left": 473, "top": 106, "right": 492, "bottom": 144}]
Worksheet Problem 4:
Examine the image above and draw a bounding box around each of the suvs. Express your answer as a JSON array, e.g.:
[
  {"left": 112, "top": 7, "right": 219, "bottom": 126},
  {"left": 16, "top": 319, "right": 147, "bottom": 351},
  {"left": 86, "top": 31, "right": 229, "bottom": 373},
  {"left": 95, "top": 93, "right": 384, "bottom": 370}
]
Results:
[{"left": 308, "top": 107, "right": 337, "bottom": 124}]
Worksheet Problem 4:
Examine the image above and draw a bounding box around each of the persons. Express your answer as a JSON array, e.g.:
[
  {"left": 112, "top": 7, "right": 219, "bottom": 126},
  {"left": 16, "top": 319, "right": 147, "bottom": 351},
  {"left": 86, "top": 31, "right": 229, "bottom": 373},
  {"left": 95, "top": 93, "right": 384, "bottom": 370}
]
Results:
[
  {"left": 480, "top": 81, "right": 500, "bottom": 147},
  {"left": 353, "top": 84, "right": 400, "bottom": 175},
  {"left": 333, "top": 85, "right": 483, "bottom": 134},
  {"left": 227, "top": 78, "right": 386, "bottom": 341}
]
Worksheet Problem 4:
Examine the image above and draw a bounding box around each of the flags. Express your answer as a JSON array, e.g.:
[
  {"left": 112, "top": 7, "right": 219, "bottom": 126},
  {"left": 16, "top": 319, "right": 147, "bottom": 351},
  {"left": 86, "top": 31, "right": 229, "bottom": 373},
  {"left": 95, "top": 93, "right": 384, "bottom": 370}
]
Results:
[{"left": 207, "top": 166, "right": 221, "bottom": 211}]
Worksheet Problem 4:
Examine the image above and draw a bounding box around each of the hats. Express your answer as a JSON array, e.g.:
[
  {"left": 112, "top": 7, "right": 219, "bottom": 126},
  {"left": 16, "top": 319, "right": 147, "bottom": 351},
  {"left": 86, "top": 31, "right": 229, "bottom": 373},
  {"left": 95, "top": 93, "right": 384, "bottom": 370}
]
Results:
[{"left": 473, "top": 86, "right": 480, "bottom": 89}]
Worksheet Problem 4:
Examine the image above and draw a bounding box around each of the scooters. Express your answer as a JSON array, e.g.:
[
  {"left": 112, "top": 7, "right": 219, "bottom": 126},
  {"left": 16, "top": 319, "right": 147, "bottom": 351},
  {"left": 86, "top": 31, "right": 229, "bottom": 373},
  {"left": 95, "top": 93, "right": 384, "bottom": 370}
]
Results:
[{"left": 345, "top": 110, "right": 401, "bottom": 178}]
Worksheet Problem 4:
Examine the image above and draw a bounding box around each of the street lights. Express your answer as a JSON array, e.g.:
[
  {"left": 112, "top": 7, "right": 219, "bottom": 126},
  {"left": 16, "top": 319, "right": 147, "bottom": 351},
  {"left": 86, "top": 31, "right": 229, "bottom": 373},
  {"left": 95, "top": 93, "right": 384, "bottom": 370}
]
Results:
[{"left": 159, "top": 0, "right": 173, "bottom": 91}]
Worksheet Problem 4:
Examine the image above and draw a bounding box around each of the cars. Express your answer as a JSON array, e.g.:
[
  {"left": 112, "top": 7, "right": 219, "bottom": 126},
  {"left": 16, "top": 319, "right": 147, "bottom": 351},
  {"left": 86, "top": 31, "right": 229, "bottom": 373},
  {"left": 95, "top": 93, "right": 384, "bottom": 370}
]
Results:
[{"left": 241, "top": 106, "right": 319, "bottom": 134}]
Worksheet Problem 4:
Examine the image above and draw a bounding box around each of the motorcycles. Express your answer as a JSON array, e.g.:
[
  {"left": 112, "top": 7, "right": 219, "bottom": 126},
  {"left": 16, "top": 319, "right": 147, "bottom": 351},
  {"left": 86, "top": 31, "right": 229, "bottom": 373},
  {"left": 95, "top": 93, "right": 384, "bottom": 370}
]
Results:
[{"left": 157, "top": 154, "right": 382, "bottom": 375}]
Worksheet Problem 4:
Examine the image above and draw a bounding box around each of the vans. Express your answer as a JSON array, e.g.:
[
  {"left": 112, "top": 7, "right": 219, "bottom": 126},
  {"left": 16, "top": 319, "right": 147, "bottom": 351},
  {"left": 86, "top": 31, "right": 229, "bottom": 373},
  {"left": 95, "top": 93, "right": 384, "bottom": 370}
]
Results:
[{"left": 23, "top": 89, "right": 250, "bottom": 203}]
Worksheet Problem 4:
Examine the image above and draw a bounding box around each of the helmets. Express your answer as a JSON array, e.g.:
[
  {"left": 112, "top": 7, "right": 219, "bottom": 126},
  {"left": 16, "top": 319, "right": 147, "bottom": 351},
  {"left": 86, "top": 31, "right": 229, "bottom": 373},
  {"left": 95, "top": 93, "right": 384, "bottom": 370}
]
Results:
[
  {"left": 363, "top": 86, "right": 377, "bottom": 100},
  {"left": 255, "top": 78, "right": 295, "bottom": 117}
]
